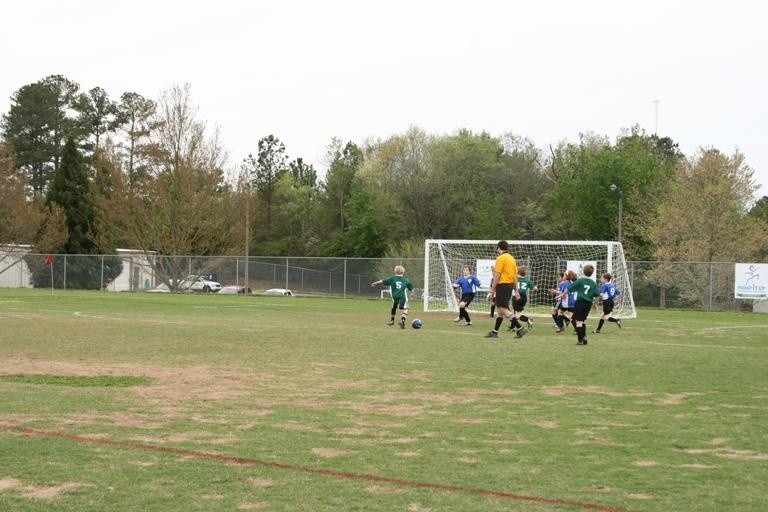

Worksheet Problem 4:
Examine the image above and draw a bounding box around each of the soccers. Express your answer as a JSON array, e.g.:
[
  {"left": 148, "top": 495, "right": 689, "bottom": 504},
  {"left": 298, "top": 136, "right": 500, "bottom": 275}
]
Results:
[{"left": 412, "top": 319, "right": 422, "bottom": 329}]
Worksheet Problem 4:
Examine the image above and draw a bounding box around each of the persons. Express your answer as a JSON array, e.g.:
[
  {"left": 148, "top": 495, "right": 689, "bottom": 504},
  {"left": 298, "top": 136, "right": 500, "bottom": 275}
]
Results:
[
  {"left": 548, "top": 265, "right": 621, "bottom": 346},
  {"left": 484, "top": 240, "right": 538, "bottom": 337},
  {"left": 371, "top": 266, "right": 413, "bottom": 328},
  {"left": 452, "top": 267, "right": 481, "bottom": 325}
]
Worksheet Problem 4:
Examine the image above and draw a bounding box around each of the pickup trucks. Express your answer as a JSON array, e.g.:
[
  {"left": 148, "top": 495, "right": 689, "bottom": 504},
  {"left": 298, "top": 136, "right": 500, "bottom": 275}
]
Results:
[{"left": 169, "top": 275, "right": 222, "bottom": 294}]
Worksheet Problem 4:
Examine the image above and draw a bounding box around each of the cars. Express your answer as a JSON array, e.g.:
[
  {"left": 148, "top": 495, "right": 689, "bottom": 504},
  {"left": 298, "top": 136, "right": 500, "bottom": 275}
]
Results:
[
  {"left": 264, "top": 288, "right": 293, "bottom": 296},
  {"left": 216, "top": 284, "right": 252, "bottom": 294}
]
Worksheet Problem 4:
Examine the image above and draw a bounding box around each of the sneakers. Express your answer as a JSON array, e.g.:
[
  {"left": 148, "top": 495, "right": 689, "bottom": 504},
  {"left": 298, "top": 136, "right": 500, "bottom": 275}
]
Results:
[
  {"left": 508, "top": 318, "right": 533, "bottom": 339},
  {"left": 485, "top": 331, "right": 497, "bottom": 337},
  {"left": 553, "top": 319, "right": 577, "bottom": 335},
  {"left": 617, "top": 319, "right": 621, "bottom": 327},
  {"left": 388, "top": 320, "right": 405, "bottom": 328},
  {"left": 577, "top": 336, "right": 587, "bottom": 345},
  {"left": 454, "top": 316, "right": 472, "bottom": 325},
  {"left": 592, "top": 329, "right": 600, "bottom": 333}
]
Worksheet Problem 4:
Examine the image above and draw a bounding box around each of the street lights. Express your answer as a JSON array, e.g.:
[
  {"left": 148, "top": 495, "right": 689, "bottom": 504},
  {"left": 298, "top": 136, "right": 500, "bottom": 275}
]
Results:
[{"left": 610, "top": 183, "right": 623, "bottom": 309}]
